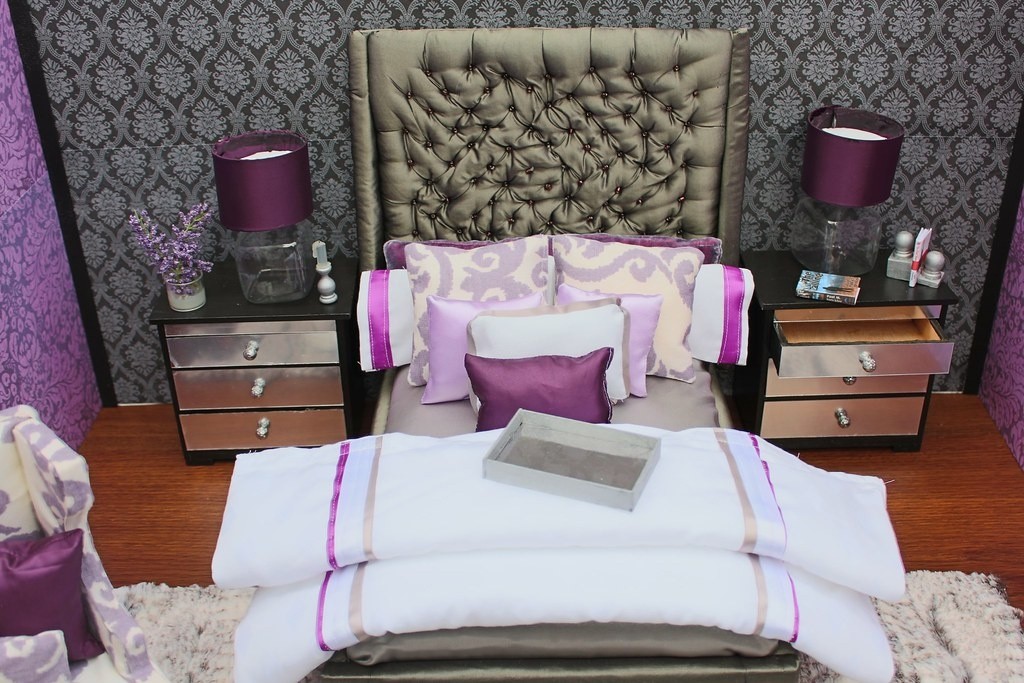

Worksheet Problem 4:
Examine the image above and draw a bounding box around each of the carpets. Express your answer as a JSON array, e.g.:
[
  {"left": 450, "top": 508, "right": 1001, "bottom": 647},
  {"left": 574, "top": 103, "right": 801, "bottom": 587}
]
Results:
[{"left": 116, "top": 570, "right": 1024, "bottom": 683}]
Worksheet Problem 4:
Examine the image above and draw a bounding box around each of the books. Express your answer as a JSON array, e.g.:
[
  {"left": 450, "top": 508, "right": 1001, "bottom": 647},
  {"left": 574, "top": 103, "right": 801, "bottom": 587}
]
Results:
[{"left": 795, "top": 270, "right": 860, "bottom": 306}]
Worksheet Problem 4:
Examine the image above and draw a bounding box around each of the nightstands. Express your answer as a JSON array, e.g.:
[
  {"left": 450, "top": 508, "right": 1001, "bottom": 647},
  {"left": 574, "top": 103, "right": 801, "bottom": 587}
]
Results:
[
  {"left": 148, "top": 266, "right": 357, "bottom": 468},
  {"left": 732, "top": 250, "right": 959, "bottom": 453}
]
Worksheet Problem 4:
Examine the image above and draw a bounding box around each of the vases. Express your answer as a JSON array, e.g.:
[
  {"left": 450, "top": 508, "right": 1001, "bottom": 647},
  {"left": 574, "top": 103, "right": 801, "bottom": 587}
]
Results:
[{"left": 162, "top": 270, "right": 206, "bottom": 312}]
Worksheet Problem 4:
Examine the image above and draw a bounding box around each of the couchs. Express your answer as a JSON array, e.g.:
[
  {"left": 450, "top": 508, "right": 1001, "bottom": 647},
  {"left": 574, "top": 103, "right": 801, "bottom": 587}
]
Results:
[{"left": 0, "top": 404, "right": 169, "bottom": 683}]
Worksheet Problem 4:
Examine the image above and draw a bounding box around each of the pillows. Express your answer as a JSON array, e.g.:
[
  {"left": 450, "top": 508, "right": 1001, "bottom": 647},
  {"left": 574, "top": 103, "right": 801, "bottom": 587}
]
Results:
[
  {"left": 383, "top": 237, "right": 723, "bottom": 269},
  {"left": 0, "top": 528, "right": 106, "bottom": 660},
  {"left": 555, "top": 284, "right": 664, "bottom": 398},
  {"left": 420, "top": 286, "right": 542, "bottom": 405},
  {"left": 357, "top": 264, "right": 754, "bottom": 372},
  {"left": 551, "top": 235, "right": 705, "bottom": 384},
  {"left": 467, "top": 297, "right": 627, "bottom": 417},
  {"left": 465, "top": 348, "right": 613, "bottom": 433},
  {"left": 404, "top": 234, "right": 549, "bottom": 387},
  {"left": 0, "top": 630, "right": 72, "bottom": 683}
]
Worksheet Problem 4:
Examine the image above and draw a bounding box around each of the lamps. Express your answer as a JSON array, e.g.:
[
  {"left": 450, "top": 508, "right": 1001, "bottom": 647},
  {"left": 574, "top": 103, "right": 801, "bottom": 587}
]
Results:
[
  {"left": 211, "top": 131, "right": 313, "bottom": 305},
  {"left": 790, "top": 105, "right": 904, "bottom": 275}
]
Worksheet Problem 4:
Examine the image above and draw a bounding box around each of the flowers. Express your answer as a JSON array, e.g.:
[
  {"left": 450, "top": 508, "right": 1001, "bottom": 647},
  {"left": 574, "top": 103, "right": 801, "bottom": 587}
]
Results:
[{"left": 129, "top": 204, "right": 216, "bottom": 296}]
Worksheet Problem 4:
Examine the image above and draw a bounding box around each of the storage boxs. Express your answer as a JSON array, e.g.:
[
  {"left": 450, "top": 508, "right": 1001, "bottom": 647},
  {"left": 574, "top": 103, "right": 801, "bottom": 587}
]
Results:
[{"left": 482, "top": 408, "right": 661, "bottom": 512}]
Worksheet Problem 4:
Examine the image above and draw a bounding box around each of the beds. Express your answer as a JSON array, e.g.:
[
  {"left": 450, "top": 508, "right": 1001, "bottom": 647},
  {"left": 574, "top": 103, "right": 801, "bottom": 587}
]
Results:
[{"left": 212, "top": 28, "right": 908, "bottom": 683}]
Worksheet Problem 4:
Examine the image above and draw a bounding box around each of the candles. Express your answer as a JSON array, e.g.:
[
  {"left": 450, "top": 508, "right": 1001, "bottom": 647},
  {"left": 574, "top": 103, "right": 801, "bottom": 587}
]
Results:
[{"left": 312, "top": 240, "right": 328, "bottom": 269}]
